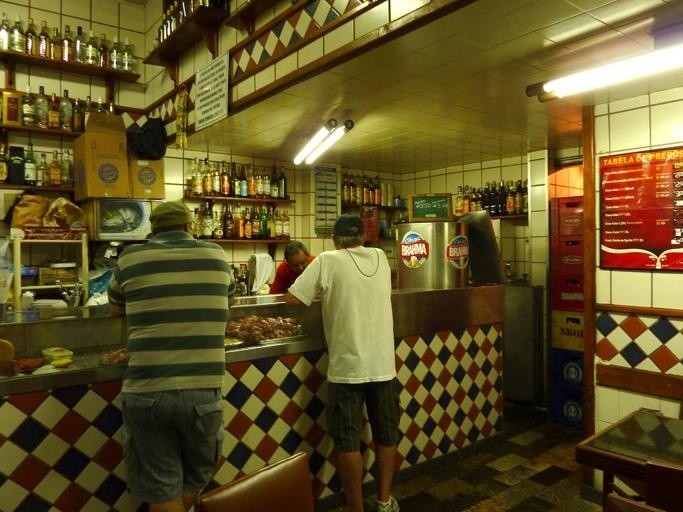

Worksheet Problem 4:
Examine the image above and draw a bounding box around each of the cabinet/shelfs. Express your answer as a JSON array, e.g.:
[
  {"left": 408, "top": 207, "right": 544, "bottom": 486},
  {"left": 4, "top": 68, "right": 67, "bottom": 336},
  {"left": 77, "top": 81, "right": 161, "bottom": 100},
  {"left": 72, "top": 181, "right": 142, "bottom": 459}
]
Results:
[
  {"left": 0, "top": 50, "right": 140, "bottom": 193},
  {"left": 341, "top": 203, "right": 407, "bottom": 240},
  {"left": 8, "top": 234, "right": 89, "bottom": 305},
  {"left": 184, "top": 196, "right": 297, "bottom": 243}
]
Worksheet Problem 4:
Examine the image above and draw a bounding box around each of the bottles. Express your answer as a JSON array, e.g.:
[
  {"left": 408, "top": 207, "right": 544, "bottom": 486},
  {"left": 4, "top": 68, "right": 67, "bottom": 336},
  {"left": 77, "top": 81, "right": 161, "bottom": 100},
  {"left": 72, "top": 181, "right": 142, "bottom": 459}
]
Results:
[
  {"left": 0, "top": 143, "right": 72, "bottom": 187},
  {"left": 191, "top": 201, "right": 290, "bottom": 240},
  {"left": 454, "top": 179, "right": 529, "bottom": 217},
  {"left": 190, "top": 155, "right": 287, "bottom": 199},
  {"left": 341, "top": 172, "right": 382, "bottom": 205},
  {"left": 228, "top": 263, "right": 249, "bottom": 295},
  {"left": 21, "top": 85, "right": 114, "bottom": 133},
  {"left": 0, "top": 12, "right": 135, "bottom": 75},
  {"left": 21, "top": 290, "right": 37, "bottom": 311},
  {"left": 158, "top": 1, "right": 210, "bottom": 47}
]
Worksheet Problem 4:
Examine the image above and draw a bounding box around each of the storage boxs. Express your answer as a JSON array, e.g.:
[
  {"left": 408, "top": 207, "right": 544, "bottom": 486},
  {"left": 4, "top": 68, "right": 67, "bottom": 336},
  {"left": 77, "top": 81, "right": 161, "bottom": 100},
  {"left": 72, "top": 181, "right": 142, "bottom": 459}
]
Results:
[
  {"left": 27, "top": 263, "right": 80, "bottom": 286},
  {"left": 131, "top": 145, "right": 165, "bottom": 200},
  {"left": 73, "top": 112, "right": 130, "bottom": 201}
]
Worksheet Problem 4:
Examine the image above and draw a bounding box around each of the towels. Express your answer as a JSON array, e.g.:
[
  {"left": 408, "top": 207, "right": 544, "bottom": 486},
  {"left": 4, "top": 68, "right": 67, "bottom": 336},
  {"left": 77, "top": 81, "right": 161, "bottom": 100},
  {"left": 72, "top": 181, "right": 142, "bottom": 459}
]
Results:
[
  {"left": 251, "top": 252, "right": 276, "bottom": 294},
  {"left": 83, "top": 290, "right": 111, "bottom": 307}
]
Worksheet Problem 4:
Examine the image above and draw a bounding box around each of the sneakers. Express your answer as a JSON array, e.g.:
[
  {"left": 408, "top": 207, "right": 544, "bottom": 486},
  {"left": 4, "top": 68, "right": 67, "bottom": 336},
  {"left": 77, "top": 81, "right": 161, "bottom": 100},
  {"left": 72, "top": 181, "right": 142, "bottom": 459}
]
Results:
[{"left": 377, "top": 494, "right": 400, "bottom": 512}]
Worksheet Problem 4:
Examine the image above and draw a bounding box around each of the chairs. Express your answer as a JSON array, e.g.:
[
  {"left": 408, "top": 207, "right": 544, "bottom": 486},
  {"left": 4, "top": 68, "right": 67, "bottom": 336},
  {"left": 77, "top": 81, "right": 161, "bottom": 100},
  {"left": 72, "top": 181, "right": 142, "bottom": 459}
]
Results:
[{"left": 194, "top": 451, "right": 317, "bottom": 512}]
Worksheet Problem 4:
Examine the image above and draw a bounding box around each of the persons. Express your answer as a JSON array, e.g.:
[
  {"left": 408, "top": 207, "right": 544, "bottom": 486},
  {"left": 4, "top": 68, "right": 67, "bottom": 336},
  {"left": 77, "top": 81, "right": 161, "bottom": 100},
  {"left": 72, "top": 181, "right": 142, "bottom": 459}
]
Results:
[
  {"left": 270, "top": 241, "right": 317, "bottom": 294},
  {"left": 285, "top": 214, "right": 401, "bottom": 512},
  {"left": 106, "top": 201, "right": 237, "bottom": 511}
]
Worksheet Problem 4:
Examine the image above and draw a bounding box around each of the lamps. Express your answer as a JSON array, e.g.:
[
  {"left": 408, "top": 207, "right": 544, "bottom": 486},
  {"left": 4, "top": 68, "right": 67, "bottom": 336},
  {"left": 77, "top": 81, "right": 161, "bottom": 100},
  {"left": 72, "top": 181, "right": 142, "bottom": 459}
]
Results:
[
  {"left": 305, "top": 120, "right": 355, "bottom": 166},
  {"left": 526, "top": 43, "right": 683, "bottom": 102},
  {"left": 294, "top": 118, "right": 337, "bottom": 166}
]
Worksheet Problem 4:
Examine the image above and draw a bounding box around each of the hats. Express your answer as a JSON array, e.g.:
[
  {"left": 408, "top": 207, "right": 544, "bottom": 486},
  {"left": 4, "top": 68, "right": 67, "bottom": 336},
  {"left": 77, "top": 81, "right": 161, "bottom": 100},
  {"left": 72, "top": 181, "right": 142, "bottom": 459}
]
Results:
[
  {"left": 334, "top": 214, "right": 364, "bottom": 236},
  {"left": 149, "top": 200, "right": 193, "bottom": 231}
]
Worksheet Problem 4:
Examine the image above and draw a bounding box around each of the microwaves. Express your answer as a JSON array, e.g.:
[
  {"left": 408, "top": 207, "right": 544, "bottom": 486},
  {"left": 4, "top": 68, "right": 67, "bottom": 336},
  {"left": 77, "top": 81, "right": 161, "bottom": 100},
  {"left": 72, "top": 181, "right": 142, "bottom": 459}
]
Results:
[{"left": 84, "top": 197, "right": 152, "bottom": 242}]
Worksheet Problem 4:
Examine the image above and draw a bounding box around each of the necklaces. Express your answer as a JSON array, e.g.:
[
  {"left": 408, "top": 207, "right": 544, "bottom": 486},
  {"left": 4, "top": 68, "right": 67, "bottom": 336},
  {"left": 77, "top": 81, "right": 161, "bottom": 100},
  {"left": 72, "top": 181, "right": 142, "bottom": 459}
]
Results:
[{"left": 345, "top": 247, "right": 380, "bottom": 278}]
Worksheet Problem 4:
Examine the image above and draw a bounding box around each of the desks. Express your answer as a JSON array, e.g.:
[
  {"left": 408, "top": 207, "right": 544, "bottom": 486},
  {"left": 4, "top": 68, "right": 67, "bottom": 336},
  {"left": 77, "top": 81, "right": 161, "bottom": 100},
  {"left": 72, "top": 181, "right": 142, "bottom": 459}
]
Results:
[{"left": 575, "top": 407, "right": 683, "bottom": 512}]
